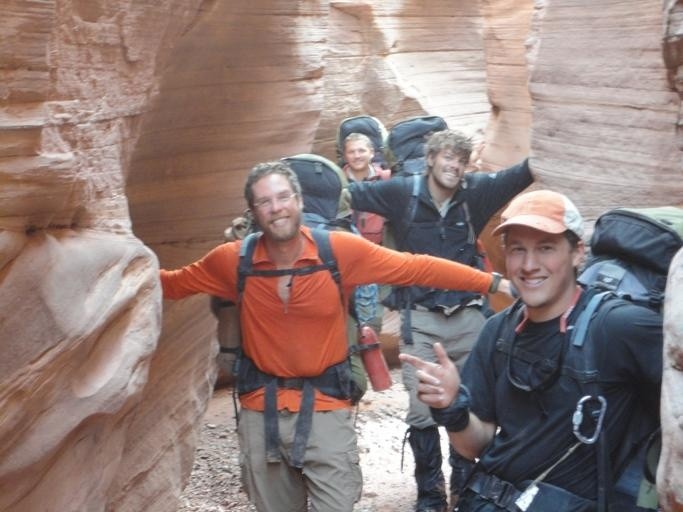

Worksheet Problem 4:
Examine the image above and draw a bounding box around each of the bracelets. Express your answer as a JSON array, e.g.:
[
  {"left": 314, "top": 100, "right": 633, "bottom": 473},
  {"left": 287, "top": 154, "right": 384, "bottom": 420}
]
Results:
[{"left": 428, "top": 384, "right": 473, "bottom": 432}]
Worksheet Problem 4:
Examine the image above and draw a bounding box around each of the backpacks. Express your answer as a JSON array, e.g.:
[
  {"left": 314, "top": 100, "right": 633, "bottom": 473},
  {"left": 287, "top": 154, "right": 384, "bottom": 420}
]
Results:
[
  {"left": 386, "top": 116, "right": 492, "bottom": 345},
  {"left": 454, "top": 206, "right": 683, "bottom": 512},
  {"left": 335, "top": 114, "right": 389, "bottom": 171},
  {"left": 213, "top": 154, "right": 381, "bottom": 469}
]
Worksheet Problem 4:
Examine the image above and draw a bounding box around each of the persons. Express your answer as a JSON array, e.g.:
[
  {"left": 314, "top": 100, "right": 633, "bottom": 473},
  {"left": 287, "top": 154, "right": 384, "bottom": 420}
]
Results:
[
  {"left": 393, "top": 188, "right": 661, "bottom": 511},
  {"left": 334, "top": 134, "right": 393, "bottom": 342},
  {"left": 343, "top": 130, "right": 535, "bottom": 510},
  {"left": 159, "top": 161, "right": 517, "bottom": 511}
]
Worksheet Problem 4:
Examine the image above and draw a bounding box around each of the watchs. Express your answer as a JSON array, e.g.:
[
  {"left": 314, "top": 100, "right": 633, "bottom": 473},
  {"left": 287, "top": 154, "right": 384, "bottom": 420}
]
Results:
[{"left": 489, "top": 268, "right": 504, "bottom": 295}]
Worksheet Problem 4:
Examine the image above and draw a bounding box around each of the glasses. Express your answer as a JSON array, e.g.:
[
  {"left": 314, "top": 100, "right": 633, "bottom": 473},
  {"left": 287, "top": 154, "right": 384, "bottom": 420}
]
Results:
[
  {"left": 252, "top": 192, "right": 298, "bottom": 208},
  {"left": 503, "top": 343, "right": 564, "bottom": 395}
]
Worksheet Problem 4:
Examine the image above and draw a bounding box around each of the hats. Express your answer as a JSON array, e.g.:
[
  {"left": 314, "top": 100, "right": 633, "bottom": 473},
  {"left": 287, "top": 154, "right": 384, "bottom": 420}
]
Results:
[{"left": 490, "top": 189, "right": 584, "bottom": 240}]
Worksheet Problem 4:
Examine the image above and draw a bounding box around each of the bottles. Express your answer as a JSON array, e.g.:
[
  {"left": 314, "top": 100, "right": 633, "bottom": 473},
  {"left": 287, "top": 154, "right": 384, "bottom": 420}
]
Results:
[
  {"left": 475, "top": 238, "right": 493, "bottom": 274},
  {"left": 356, "top": 323, "right": 394, "bottom": 394}
]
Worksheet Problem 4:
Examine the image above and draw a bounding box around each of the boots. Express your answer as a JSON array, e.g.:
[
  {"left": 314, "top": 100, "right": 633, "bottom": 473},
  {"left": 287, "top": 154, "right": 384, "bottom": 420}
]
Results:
[{"left": 401, "top": 424, "right": 447, "bottom": 512}]
